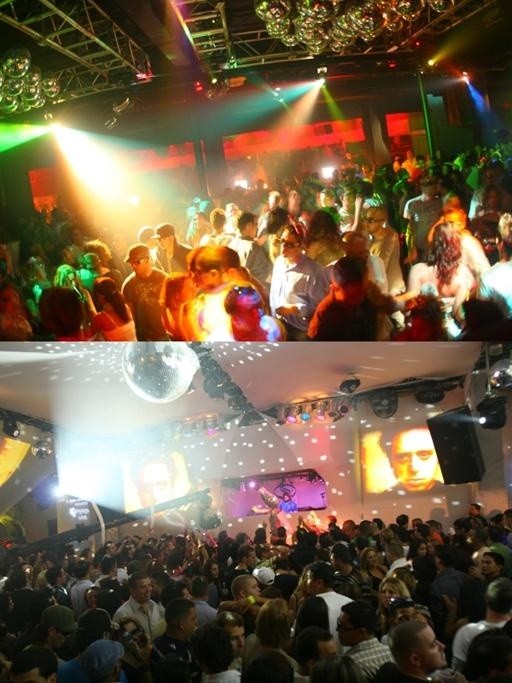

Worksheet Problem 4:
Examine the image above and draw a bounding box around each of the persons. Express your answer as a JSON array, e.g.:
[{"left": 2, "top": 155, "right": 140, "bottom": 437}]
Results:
[{"left": 378, "top": 421, "right": 446, "bottom": 494}]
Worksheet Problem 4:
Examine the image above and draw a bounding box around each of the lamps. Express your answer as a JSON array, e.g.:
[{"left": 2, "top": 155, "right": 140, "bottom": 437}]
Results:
[{"left": 0, "top": 341, "right": 512, "bottom": 459}]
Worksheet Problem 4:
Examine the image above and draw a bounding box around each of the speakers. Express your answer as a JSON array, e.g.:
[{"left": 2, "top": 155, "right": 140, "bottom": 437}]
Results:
[
  {"left": 95, "top": 463, "right": 125, "bottom": 522},
  {"left": 427, "top": 405, "right": 486, "bottom": 485}
]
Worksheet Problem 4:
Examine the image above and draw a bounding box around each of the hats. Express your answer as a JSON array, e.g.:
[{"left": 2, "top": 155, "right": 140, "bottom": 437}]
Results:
[
  {"left": 76, "top": 608, "right": 119, "bottom": 631},
  {"left": 150, "top": 223, "right": 174, "bottom": 239},
  {"left": 39, "top": 604, "right": 78, "bottom": 632},
  {"left": 122, "top": 244, "right": 149, "bottom": 262},
  {"left": 251, "top": 566, "right": 274, "bottom": 586},
  {"left": 80, "top": 638, "right": 125, "bottom": 675}
]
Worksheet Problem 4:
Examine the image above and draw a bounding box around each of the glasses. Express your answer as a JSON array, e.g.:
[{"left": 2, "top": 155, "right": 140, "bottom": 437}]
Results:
[
  {"left": 364, "top": 215, "right": 381, "bottom": 222},
  {"left": 280, "top": 237, "right": 300, "bottom": 248},
  {"left": 134, "top": 257, "right": 144, "bottom": 264},
  {"left": 336, "top": 617, "right": 357, "bottom": 631}
]
